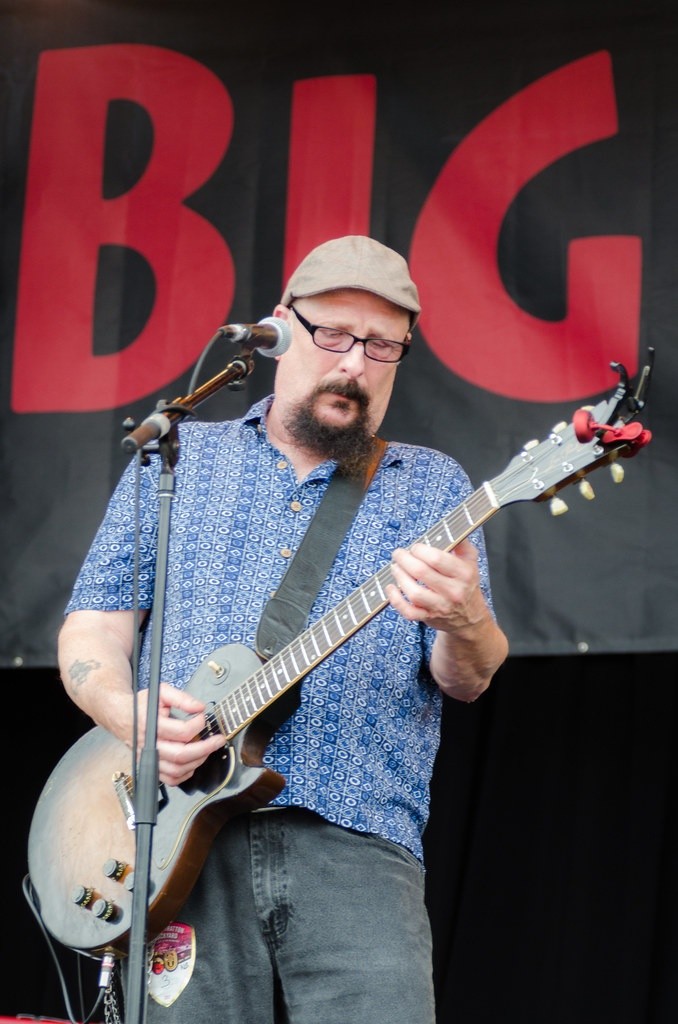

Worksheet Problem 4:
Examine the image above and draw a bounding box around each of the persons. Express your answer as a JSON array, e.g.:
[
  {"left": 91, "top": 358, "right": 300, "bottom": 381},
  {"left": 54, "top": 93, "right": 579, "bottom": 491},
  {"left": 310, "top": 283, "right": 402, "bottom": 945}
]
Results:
[{"left": 57, "top": 229, "right": 507, "bottom": 1023}]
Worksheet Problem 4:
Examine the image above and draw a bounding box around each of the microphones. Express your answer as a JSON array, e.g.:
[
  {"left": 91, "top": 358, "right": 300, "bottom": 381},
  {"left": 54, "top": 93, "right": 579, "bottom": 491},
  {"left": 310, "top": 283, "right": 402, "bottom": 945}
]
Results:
[{"left": 219, "top": 316, "right": 291, "bottom": 357}]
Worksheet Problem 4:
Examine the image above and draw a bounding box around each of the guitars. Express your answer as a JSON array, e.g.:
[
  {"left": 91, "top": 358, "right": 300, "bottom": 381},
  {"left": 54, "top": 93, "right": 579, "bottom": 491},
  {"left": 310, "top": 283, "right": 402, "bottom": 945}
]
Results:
[{"left": 24, "top": 345, "right": 661, "bottom": 961}]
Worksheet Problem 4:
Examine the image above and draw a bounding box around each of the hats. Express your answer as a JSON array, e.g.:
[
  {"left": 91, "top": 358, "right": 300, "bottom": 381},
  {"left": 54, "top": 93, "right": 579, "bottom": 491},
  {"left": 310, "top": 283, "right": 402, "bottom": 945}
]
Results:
[{"left": 280, "top": 235, "right": 423, "bottom": 333}]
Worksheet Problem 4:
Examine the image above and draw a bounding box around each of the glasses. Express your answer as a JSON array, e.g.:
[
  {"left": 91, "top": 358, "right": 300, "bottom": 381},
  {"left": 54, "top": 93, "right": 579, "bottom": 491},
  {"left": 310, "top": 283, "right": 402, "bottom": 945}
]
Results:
[{"left": 289, "top": 303, "right": 413, "bottom": 364}]
